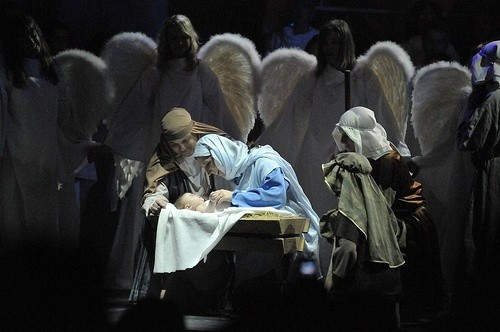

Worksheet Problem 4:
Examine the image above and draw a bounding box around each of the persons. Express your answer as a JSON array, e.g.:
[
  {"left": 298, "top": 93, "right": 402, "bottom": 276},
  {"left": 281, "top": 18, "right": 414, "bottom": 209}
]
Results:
[
  {"left": 332, "top": 105, "right": 441, "bottom": 301},
  {"left": 250, "top": 19, "right": 419, "bottom": 282},
  {"left": 175, "top": 191, "right": 232, "bottom": 212},
  {"left": 194, "top": 134, "right": 320, "bottom": 264},
  {"left": 0, "top": 15, "right": 110, "bottom": 247},
  {"left": 129, "top": 107, "right": 235, "bottom": 305},
  {"left": 88, "top": 14, "right": 241, "bottom": 293},
  {"left": 407, "top": 40, "right": 500, "bottom": 283},
  {"left": 318, "top": 151, "right": 405, "bottom": 299}
]
